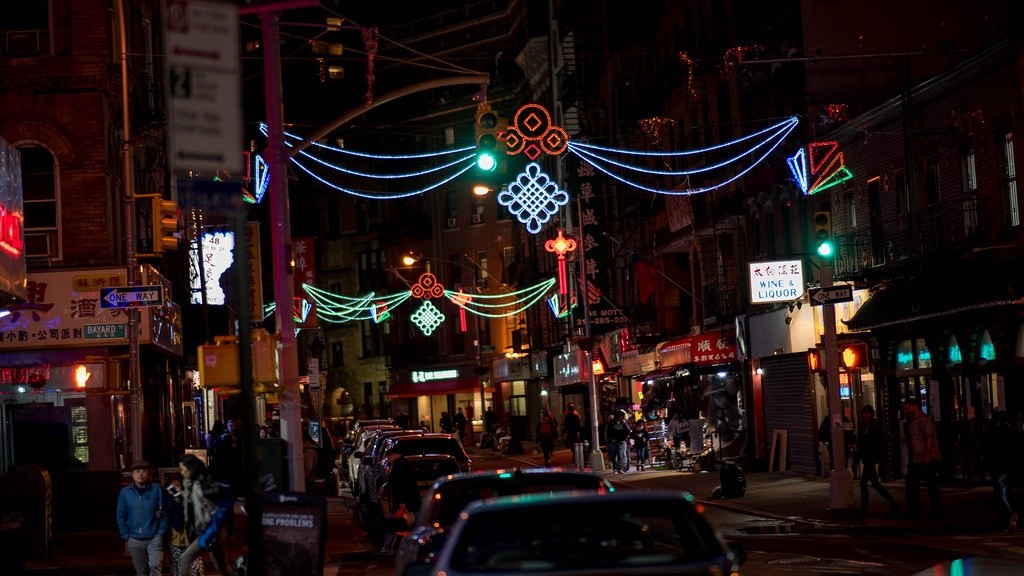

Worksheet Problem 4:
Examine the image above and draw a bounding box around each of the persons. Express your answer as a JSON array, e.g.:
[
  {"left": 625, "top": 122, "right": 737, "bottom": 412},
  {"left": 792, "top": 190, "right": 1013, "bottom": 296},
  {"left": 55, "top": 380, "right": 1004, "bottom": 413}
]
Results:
[
  {"left": 586, "top": 402, "right": 650, "bottom": 474},
  {"left": 534, "top": 402, "right": 580, "bottom": 467},
  {"left": 116, "top": 454, "right": 236, "bottom": 576},
  {"left": 481, "top": 407, "right": 497, "bottom": 433},
  {"left": 981, "top": 411, "right": 1019, "bottom": 532},
  {"left": 374, "top": 448, "right": 420, "bottom": 556},
  {"left": 853, "top": 404, "right": 903, "bottom": 514},
  {"left": 818, "top": 400, "right": 856, "bottom": 470},
  {"left": 396, "top": 410, "right": 412, "bottom": 429},
  {"left": 209, "top": 418, "right": 325, "bottom": 481},
  {"left": 898, "top": 399, "right": 943, "bottom": 519},
  {"left": 439, "top": 408, "right": 466, "bottom": 446}
]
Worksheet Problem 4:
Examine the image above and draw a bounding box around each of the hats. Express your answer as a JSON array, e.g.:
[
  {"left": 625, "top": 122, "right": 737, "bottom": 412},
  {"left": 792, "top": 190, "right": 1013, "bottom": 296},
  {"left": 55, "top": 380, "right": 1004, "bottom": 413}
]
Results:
[{"left": 131, "top": 459, "right": 150, "bottom": 469}]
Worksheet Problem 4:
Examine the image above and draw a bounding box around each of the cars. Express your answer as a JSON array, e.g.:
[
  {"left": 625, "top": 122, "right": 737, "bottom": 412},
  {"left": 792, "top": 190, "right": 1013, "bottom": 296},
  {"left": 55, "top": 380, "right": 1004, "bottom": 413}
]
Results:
[
  {"left": 306, "top": 416, "right": 630, "bottom": 576},
  {"left": 399, "top": 487, "right": 749, "bottom": 576}
]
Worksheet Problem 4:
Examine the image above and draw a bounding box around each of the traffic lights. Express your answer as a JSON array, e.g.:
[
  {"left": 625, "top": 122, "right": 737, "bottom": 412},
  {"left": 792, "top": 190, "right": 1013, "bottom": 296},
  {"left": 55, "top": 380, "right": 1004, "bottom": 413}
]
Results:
[
  {"left": 840, "top": 341, "right": 870, "bottom": 371},
  {"left": 494, "top": 117, "right": 509, "bottom": 176},
  {"left": 251, "top": 335, "right": 278, "bottom": 385},
  {"left": 814, "top": 211, "right": 831, "bottom": 260},
  {"left": 474, "top": 104, "right": 498, "bottom": 176},
  {"left": 400, "top": 254, "right": 416, "bottom": 290},
  {"left": 152, "top": 198, "right": 179, "bottom": 253},
  {"left": 807, "top": 348, "right": 827, "bottom": 373}
]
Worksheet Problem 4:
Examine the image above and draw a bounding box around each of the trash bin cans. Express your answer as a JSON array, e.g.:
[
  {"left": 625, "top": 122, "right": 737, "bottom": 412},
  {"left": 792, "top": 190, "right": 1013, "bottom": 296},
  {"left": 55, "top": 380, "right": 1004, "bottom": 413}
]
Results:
[
  {"left": 0, "top": 464, "right": 54, "bottom": 558},
  {"left": 240, "top": 493, "right": 327, "bottom": 576}
]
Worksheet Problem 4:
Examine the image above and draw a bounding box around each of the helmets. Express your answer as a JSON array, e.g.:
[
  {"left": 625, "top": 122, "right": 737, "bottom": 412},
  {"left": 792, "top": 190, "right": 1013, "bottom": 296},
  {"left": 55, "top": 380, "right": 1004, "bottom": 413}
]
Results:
[{"left": 567, "top": 401, "right": 575, "bottom": 409}]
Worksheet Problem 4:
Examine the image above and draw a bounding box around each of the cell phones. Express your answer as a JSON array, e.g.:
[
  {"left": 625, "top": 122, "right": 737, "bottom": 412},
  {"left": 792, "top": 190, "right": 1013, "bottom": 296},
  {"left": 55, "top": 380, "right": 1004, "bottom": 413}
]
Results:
[{"left": 168, "top": 485, "right": 180, "bottom": 496}]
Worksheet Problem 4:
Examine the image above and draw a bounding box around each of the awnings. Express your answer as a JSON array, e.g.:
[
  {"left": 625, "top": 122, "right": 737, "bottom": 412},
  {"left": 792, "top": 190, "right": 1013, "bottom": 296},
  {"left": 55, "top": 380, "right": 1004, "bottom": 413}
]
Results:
[
  {"left": 849, "top": 256, "right": 1024, "bottom": 331},
  {"left": 385, "top": 375, "right": 495, "bottom": 398}
]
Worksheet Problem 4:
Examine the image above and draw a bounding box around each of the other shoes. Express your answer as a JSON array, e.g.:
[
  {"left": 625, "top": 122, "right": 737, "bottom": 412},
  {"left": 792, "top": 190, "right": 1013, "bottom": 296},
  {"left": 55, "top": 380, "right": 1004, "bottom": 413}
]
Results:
[
  {"left": 636, "top": 464, "right": 640, "bottom": 471},
  {"left": 887, "top": 505, "right": 901, "bottom": 517},
  {"left": 1009, "top": 512, "right": 1018, "bottom": 532},
  {"left": 619, "top": 469, "right": 624, "bottom": 474},
  {"left": 613, "top": 469, "right": 618, "bottom": 475},
  {"left": 899, "top": 511, "right": 920, "bottom": 520},
  {"left": 641, "top": 464, "right": 644, "bottom": 471}
]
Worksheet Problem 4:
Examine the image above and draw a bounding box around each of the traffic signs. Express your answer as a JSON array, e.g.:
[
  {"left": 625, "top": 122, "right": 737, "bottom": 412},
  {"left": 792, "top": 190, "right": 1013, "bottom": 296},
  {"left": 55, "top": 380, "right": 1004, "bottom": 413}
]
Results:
[
  {"left": 163, "top": 63, "right": 245, "bottom": 177},
  {"left": 156, "top": 0, "right": 240, "bottom": 75},
  {"left": 98, "top": 284, "right": 163, "bottom": 310},
  {"left": 807, "top": 284, "right": 854, "bottom": 308}
]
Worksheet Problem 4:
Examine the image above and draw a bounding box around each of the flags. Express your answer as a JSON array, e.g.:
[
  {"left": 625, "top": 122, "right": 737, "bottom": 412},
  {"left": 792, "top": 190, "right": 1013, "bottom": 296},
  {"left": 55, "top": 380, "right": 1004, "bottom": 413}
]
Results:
[
  {"left": 632, "top": 251, "right": 659, "bottom": 305},
  {"left": 575, "top": 269, "right": 602, "bottom": 307}
]
